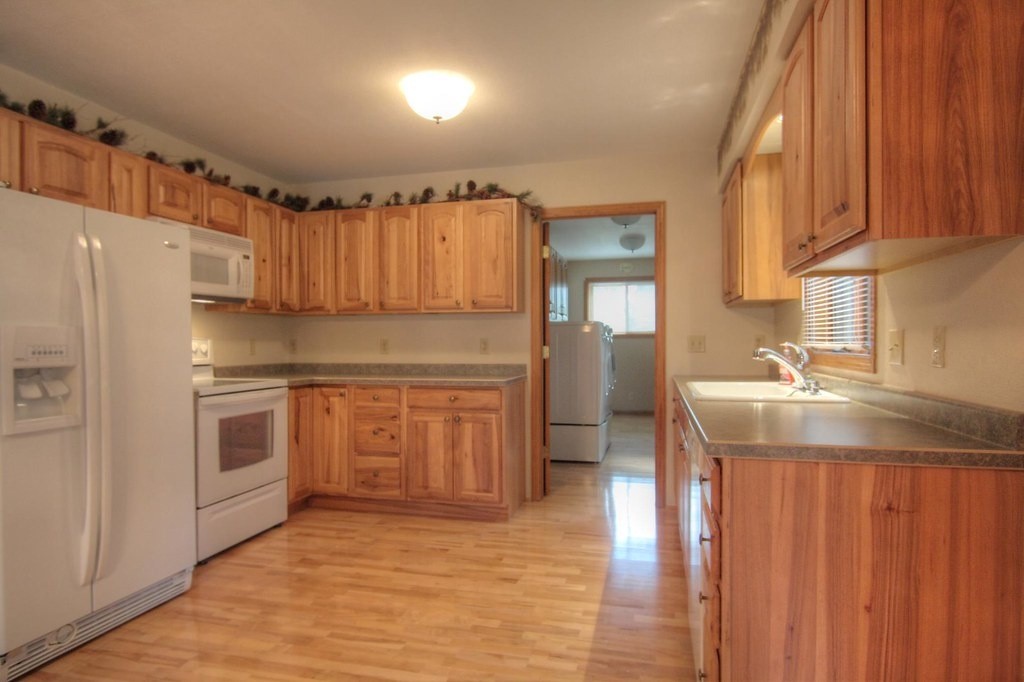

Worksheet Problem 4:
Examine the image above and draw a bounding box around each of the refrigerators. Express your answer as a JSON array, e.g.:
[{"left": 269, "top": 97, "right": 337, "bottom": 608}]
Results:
[{"left": 1, "top": 186, "right": 197, "bottom": 682}]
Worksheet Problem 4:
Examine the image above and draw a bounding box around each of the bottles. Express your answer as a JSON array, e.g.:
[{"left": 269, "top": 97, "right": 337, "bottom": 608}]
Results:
[{"left": 779, "top": 342, "right": 794, "bottom": 385}]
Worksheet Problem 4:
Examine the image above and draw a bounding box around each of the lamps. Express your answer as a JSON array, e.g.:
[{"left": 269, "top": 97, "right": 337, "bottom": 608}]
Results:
[
  {"left": 619, "top": 237, "right": 644, "bottom": 253},
  {"left": 397, "top": 67, "right": 475, "bottom": 126},
  {"left": 611, "top": 214, "right": 640, "bottom": 229}
]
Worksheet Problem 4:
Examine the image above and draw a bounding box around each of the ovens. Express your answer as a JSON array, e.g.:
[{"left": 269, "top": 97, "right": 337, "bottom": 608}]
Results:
[{"left": 195, "top": 386, "right": 289, "bottom": 566}]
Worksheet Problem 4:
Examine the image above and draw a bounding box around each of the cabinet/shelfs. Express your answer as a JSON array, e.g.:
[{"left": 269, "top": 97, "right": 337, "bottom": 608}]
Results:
[
  {"left": 550, "top": 245, "right": 568, "bottom": 322},
  {"left": 718, "top": 160, "right": 801, "bottom": 310},
  {"left": 1, "top": 108, "right": 526, "bottom": 313},
  {"left": 667, "top": 385, "right": 1024, "bottom": 682},
  {"left": 1, "top": 187, "right": 196, "bottom": 682},
  {"left": 781, "top": 1, "right": 1024, "bottom": 281},
  {"left": 287, "top": 386, "right": 524, "bottom": 523}
]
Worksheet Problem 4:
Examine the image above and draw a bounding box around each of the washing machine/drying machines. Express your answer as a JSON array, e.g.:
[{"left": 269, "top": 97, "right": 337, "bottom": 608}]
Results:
[{"left": 550, "top": 320, "right": 617, "bottom": 427}]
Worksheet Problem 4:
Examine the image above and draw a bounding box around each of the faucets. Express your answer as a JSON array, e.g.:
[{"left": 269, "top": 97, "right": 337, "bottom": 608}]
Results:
[{"left": 751, "top": 339, "right": 822, "bottom": 397}]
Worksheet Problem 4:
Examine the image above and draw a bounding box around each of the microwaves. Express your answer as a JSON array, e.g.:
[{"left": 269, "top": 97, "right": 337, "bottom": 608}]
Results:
[{"left": 148, "top": 216, "right": 254, "bottom": 304}]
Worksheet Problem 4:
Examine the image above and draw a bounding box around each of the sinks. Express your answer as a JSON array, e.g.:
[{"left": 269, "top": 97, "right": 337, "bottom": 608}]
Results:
[{"left": 685, "top": 377, "right": 850, "bottom": 405}]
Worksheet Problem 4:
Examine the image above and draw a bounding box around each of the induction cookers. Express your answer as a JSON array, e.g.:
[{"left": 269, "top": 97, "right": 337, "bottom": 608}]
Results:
[{"left": 192, "top": 337, "right": 288, "bottom": 397}]
